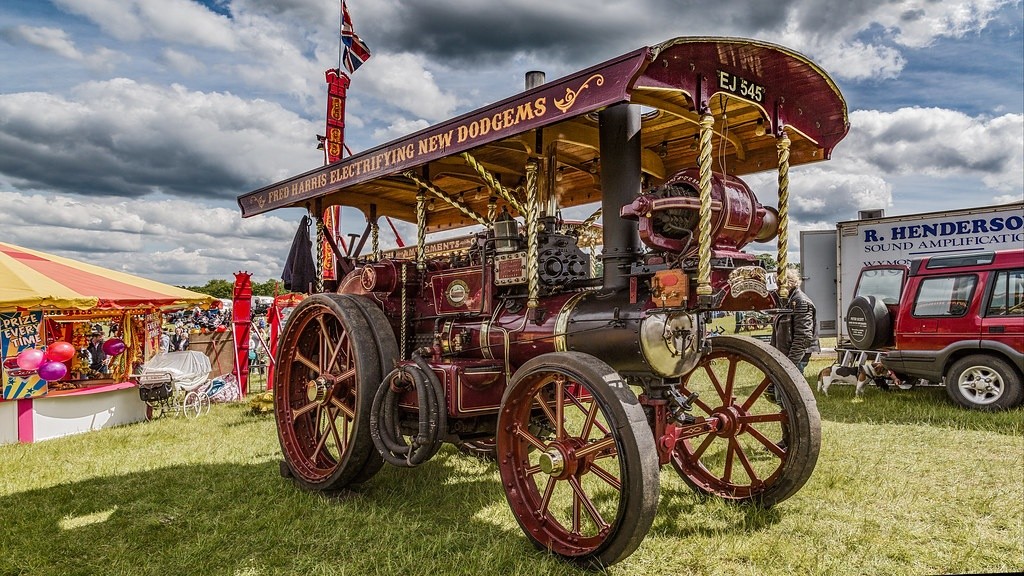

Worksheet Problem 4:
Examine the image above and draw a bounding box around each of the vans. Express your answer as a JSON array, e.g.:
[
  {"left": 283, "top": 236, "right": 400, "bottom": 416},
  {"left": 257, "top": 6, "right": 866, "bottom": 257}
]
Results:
[
  {"left": 218, "top": 298, "right": 233, "bottom": 313},
  {"left": 250, "top": 296, "right": 275, "bottom": 313}
]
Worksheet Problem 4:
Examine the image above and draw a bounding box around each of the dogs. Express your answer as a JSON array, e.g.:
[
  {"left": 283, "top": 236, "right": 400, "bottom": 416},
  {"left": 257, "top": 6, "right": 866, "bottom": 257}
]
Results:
[{"left": 816, "top": 361, "right": 892, "bottom": 398}]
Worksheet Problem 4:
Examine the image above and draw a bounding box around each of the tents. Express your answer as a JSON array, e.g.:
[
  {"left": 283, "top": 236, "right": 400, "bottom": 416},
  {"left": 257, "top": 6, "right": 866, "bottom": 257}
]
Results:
[{"left": 0, "top": 239, "right": 223, "bottom": 403}]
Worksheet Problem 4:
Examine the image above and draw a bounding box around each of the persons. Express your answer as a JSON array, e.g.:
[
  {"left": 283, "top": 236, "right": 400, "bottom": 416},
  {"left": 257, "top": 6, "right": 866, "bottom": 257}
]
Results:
[
  {"left": 763, "top": 268, "right": 821, "bottom": 454},
  {"left": 873, "top": 367, "right": 920, "bottom": 391},
  {"left": 85, "top": 300, "right": 275, "bottom": 390},
  {"left": 709, "top": 309, "right": 744, "bottom": 333}
]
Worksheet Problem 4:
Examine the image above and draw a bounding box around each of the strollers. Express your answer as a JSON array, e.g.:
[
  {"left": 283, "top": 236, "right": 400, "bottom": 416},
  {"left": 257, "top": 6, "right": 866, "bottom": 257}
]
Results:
[{"left": 129, "top": 350, "right": 211, "bottom": 422}]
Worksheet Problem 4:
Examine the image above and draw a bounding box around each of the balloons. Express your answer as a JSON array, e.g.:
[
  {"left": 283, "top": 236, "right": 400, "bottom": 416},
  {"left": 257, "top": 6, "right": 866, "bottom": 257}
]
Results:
[
  {"left": 103, "top": 339, "right": 127, "bottom": 356},
  {"left": 16, "top": 340, "right": 77, "bottom": 383},
  {"left": 190, "top": 324, "right": 227, "bottom": 334}
]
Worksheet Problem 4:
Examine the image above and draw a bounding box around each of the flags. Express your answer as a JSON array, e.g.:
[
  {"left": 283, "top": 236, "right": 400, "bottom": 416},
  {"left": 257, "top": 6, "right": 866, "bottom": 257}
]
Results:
[{"left": 340, "top": 1, "right": 372, "bottom": 74}]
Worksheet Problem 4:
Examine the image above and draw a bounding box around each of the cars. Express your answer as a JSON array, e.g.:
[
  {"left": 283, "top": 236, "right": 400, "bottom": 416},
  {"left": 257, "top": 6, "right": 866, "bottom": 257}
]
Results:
[{"left": 235, "top": 35, "right": 852, "bottom": 571}]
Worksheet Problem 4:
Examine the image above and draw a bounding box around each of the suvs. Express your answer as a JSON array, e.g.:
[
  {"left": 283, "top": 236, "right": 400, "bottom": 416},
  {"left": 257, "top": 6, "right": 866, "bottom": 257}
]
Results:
[{"left": 847, "top": 249, "right": 1024, "bottom": 410}]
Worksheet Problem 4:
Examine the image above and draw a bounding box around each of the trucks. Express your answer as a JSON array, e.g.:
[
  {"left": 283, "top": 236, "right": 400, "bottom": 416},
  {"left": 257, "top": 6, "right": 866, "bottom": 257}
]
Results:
[{"left": 800, "top": 203, "right": 1024, "bottom": 370}]
